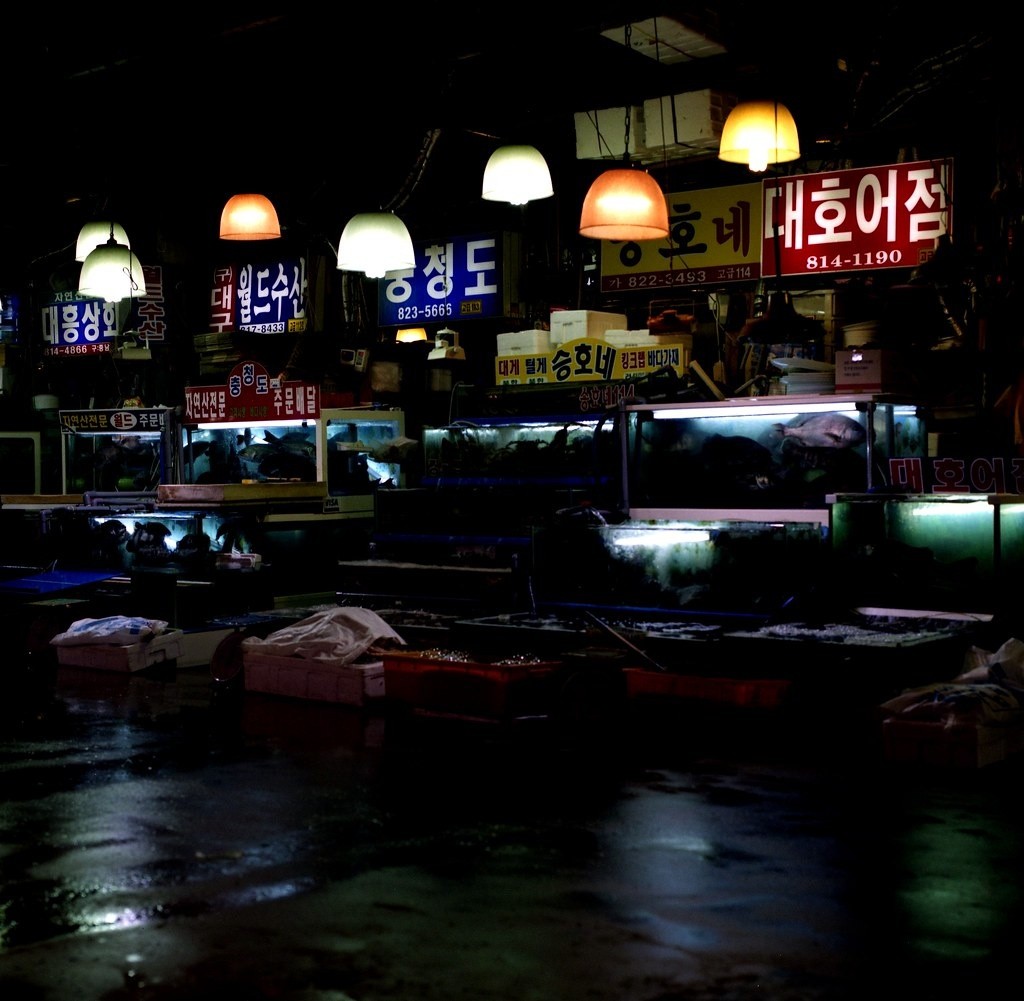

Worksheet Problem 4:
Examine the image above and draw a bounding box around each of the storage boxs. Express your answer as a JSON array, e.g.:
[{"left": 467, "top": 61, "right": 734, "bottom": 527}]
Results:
[
  {"left": 496, "top": 309, "right": 692, "bottom": 355},
  {"left": 51, "top": 399, "right": 1023, "bottom": 769},
  {"left": 834, "top": 350, "right": 913, "bottom": 387}
]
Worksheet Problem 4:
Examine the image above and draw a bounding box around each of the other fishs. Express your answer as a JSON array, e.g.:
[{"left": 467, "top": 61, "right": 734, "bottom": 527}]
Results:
[
  {"left": 183, "top": 428, "right": 316, "bottom": 478},
  {"left": 89, "top": 516, "right": 210, "bottom": 572},
  {"left": 771, "top": 414, "right": 867, "bottom": 451}
]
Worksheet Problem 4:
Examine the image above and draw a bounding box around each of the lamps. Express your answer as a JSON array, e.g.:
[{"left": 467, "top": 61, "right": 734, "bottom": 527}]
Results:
[
  {"left": 77, "top": 193, "right": 147, "bottom": 308},
  {"left": 481, "top": 146, "right": 556, "bottom": 207},
  {"left": 719, "top": 92, "right": 807, "bottom": 177},
  {"left": 427, "top": 276, "right": 466, "bottom": 363},
  {"left": 579, "top": 6, "right": 667, "bottom": 246},
  {"left": 396, "top": 329, "right": 428, "bottom": 344},
  {"left": 220, "top": 190, "right": 280, "bottom": 242},
  {"left": 75, "top": 220, "right": 130, "bottom": 263},
  {"left": 336, "top": 208, "right": 415, "bottom": 279}
]
